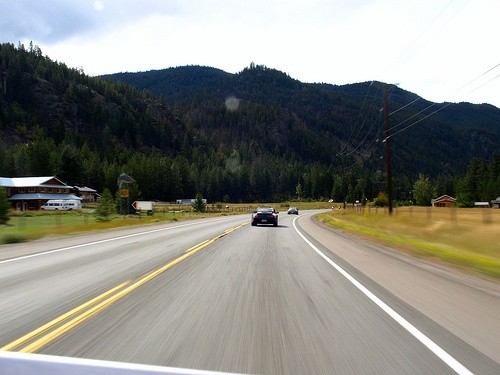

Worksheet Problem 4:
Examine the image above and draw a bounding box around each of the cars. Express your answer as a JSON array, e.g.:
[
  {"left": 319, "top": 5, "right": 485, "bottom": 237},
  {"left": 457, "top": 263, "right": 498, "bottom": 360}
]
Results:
[
  {"left": 288, "top": 207, "right": 298, "bottom": 215},
  {"left": 250, "top": 206, "right": 279, "bottom": 226}
]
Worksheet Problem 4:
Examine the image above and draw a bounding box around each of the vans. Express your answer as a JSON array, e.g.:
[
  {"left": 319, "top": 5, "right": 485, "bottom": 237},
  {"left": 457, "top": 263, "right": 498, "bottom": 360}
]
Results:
[
  {"left": 66, "top": 200, "right": 82, "bottom": 211},
  {"left": 40, "top": 200, "right": 69, "bottom": 212}
]
